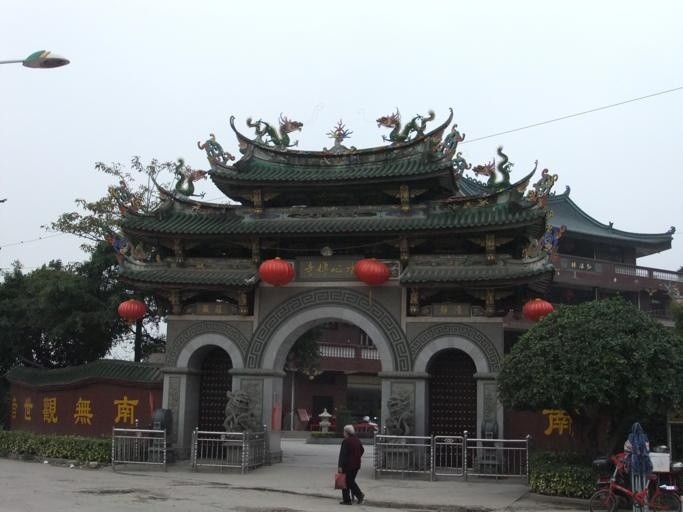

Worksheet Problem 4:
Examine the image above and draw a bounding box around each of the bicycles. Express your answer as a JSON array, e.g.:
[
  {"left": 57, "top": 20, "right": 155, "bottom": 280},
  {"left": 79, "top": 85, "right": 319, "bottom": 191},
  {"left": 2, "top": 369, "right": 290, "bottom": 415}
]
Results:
[{"left": 589, "top": 455, "right": 682, "bottom": 512}]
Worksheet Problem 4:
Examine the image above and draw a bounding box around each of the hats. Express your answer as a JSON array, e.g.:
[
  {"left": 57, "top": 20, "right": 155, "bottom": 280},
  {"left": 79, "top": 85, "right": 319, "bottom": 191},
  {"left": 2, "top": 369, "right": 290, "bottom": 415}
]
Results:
[{"left": 344, "top": 424, "right": 356, "bottom": 435}]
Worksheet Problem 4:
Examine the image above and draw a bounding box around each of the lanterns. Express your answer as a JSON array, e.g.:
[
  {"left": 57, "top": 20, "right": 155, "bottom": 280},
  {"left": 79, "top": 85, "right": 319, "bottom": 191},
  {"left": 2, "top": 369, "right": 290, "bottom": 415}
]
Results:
[
  {"left": 258, "top": 255, "right": 294, "bottom": 288},
  {"left": 117, "top": 298, "right": 147, "bottom": 330},
  {"left": 520, "top": 297, "right": 553, "bottom": 322},
  {"left": 351, "top": 254, "right": 389, "bottom": 306}
]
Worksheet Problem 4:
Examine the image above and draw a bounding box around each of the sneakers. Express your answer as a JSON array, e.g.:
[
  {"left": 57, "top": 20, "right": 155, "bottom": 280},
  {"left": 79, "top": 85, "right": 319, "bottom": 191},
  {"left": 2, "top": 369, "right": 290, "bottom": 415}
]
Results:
[
  {"left": 356, "top": 493, "right": 365, "bottom": 505},
  {"left": 340, "top": 499, "right": 353, "bottom": 505}
]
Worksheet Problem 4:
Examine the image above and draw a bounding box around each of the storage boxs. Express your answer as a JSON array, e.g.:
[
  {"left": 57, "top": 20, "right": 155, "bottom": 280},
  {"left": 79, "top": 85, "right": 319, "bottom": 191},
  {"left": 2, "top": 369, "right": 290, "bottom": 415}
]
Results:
[{"left": 649, "top": 452, "right": 671, "bottom": 473}]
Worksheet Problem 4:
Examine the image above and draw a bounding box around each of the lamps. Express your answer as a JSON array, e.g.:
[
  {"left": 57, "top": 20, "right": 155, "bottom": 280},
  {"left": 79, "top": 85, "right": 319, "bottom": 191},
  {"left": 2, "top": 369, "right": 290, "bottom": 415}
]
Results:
[{"left": 321, "top": 238, "right": 333, "bottom": 256}]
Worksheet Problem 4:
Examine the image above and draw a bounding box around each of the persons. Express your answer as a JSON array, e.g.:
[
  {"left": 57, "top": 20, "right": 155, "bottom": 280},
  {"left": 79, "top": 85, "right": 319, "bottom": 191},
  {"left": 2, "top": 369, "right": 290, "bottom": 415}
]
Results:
[
  {"left": 624, "top": 422, "right": 656, "bottom": 475},
  {"left": 333, "top": 424, "right": 364, "bottom": 504}
]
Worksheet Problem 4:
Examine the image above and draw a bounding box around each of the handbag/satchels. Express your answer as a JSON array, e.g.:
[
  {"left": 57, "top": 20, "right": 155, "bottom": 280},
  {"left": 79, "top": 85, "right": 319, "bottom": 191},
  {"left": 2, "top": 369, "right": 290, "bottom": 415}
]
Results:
[{"left": 335, "top": 472, "right": 347, "bottom": 490}]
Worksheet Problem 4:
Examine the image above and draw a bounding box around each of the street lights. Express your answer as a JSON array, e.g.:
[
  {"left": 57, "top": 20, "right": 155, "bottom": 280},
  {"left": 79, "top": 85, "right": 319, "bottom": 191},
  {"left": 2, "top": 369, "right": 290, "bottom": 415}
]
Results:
[{"left": 0, "top": 50, "right": 70, "bottom": 69}]
